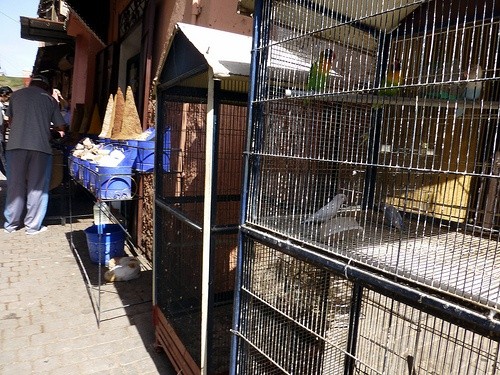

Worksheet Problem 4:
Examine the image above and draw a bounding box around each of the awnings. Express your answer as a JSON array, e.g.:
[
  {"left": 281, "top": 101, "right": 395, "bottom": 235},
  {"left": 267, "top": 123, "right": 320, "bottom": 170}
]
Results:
[
  {"left": 16, "top": 16, "right": 76, "bottom": 44},
  {"left": 32, "top": 43, "right": 71, "bottom": 74}
]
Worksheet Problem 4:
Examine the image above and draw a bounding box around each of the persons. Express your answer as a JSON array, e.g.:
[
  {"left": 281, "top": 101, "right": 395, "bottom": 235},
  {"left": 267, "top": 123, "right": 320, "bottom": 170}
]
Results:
[
  {"left": 3, "top": 76, "right": 67, "bottom": 232},
  {"left": 51, "top": 87, "right": 64, "bottom": 111},
  {"left": 0, "top": 86, "right": 14, "bottom": 177}
]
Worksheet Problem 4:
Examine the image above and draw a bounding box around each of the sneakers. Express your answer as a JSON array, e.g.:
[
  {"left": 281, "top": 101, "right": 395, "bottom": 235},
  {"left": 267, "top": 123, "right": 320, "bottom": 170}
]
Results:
[
  {"left": 4, "top": 229, "right": 16, "bottom": 233},
  {"left": 25, "top": 226, "right": 48, "bottom": 235}
]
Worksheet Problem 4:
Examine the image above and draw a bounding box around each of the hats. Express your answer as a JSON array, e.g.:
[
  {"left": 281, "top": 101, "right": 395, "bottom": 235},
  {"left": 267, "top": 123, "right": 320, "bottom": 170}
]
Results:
[{"left": 31, "top": 75, "right": 50, "bottom": 85}]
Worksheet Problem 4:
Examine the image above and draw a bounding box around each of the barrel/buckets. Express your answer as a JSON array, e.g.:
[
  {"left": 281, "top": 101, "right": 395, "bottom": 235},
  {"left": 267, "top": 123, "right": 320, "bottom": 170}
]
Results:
[
  {"left": 64, "top": 133, "right": 170, "bottom": 200},
  {"left": 83, "top": 224, "right": 125, "bottom": 265}
]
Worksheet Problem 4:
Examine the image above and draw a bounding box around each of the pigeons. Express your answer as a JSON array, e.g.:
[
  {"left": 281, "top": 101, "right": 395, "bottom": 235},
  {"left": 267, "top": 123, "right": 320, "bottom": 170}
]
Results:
[
  {"left": 300, "top": 194, "right": 347, "bottom": 223},
  {"left": 372, "top": 175, "right": 404, "bottom": 232}
]
